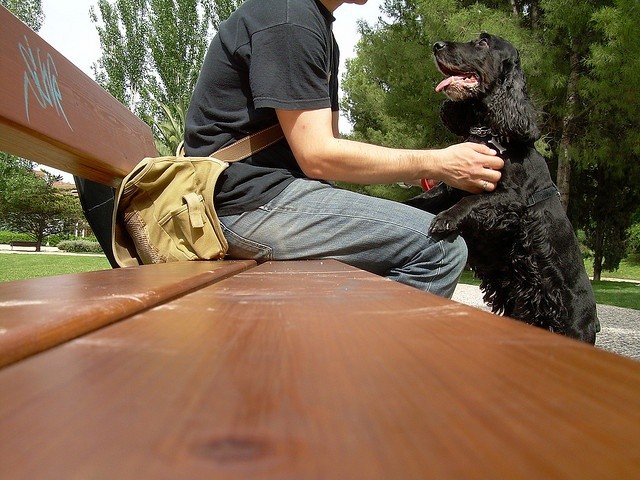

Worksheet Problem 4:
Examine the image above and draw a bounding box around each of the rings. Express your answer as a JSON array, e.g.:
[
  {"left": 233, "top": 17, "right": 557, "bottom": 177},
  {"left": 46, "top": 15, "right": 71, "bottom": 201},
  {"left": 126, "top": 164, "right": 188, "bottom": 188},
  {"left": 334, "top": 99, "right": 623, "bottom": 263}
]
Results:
[{"left": 482, "top": 181, "right": 489, "bottom": 189}]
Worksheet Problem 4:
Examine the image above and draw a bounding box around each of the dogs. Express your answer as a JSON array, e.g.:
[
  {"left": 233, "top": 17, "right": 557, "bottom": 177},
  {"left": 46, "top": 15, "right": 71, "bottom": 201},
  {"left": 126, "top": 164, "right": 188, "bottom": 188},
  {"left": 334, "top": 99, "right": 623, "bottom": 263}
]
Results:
[{"left": 400, "top": 31, "right": 601, "bottom": 347}]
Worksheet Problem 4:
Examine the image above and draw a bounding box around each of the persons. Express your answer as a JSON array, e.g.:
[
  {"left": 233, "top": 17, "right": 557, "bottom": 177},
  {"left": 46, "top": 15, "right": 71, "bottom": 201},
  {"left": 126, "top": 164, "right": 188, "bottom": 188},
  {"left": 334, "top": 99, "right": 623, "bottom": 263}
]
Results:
[{"left": 184, "top": 0, "right": 505, "bottom": 300}]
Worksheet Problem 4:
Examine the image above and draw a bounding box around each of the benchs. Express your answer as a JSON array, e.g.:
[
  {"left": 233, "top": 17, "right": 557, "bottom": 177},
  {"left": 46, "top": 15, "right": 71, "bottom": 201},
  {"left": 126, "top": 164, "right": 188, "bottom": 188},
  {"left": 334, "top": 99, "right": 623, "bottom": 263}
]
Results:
[{"left": 0, "top": 4, "right": 640, "bottom": 480}]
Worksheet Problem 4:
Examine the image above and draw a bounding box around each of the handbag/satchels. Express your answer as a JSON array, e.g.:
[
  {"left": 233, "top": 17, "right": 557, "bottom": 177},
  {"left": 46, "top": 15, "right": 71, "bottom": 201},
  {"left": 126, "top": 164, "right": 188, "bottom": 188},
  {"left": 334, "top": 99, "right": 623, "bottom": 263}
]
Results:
[{"left": 112, "top": 156, "right": 231, "bottom": 269}]
